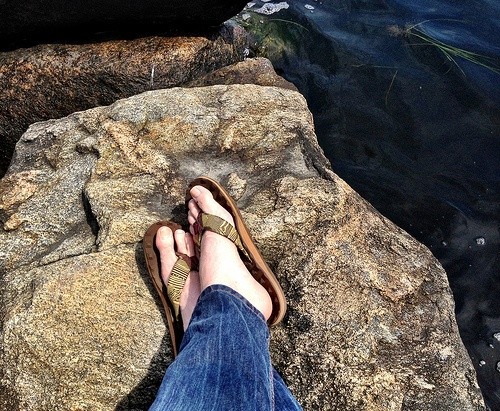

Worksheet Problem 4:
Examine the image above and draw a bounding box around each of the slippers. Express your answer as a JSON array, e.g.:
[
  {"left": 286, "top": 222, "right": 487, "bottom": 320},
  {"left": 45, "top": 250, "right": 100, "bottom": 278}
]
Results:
[{"left": 143, "top": 178, "right": 287, "bottom": 359}]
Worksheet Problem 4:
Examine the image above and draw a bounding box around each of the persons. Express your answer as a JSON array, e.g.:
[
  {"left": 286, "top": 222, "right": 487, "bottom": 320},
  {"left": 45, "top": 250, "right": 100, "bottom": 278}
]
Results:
[{"left": 143, "top": 177, "right": 302, "bottom": 411}]
